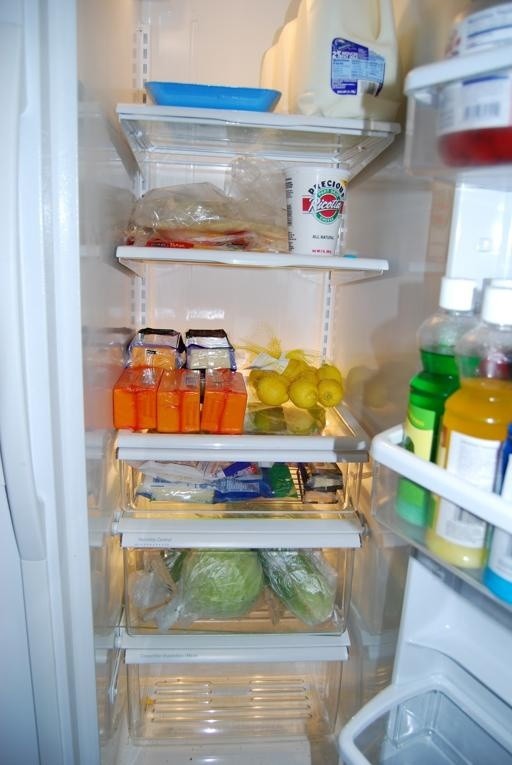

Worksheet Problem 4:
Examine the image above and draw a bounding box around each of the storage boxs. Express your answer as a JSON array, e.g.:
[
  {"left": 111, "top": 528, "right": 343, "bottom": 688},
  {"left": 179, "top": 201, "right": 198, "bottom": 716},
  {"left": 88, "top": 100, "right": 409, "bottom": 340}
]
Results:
[
  {"left": 126, "top": 660, "right": 342, "bottom": 746},
  {"left": 122, "top": 545, "right": 354, "bottom": 637}
]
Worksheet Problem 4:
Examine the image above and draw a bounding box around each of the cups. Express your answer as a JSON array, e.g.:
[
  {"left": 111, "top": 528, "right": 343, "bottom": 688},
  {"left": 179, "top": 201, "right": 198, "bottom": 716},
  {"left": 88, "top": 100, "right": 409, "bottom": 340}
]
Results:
[{"left": 282, "top": 166, "right": 351, "bottom": 256}]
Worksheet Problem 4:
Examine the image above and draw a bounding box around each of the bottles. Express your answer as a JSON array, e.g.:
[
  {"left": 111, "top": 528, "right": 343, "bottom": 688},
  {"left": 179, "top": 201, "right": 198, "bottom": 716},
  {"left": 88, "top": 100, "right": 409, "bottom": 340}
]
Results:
[{"left": 396, "top": 276, "right": 512, "bottom": 612}]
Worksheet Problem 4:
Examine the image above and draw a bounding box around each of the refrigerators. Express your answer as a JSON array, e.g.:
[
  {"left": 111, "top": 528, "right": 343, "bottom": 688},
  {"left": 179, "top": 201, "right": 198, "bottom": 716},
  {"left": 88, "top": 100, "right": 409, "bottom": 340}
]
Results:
[{"left": 1, "top": 0, "right": 512, "bottom": 765}]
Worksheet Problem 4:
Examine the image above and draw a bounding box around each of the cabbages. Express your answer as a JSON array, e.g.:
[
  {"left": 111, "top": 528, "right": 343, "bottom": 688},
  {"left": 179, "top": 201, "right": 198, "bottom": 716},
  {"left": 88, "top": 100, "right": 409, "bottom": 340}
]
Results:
[{"left": 180, "top": 551, "right": 261, "bottom": 611}]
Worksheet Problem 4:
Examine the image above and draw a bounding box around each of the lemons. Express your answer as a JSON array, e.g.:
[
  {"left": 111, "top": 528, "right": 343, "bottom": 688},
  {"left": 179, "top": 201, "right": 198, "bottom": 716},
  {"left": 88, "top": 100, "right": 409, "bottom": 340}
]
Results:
[{"left": 250, "top": 344, "right": 348, "bottom": 407}]
